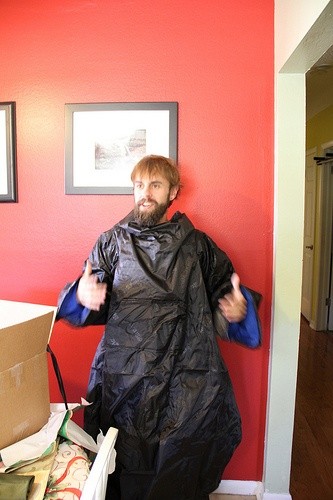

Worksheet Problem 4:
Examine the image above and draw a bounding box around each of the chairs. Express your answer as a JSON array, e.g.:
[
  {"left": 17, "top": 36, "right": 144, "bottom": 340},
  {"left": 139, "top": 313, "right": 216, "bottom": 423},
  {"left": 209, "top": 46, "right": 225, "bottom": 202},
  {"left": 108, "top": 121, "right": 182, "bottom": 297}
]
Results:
[{"left": 80, "top": 427, "right": 119, "bottom": 500}]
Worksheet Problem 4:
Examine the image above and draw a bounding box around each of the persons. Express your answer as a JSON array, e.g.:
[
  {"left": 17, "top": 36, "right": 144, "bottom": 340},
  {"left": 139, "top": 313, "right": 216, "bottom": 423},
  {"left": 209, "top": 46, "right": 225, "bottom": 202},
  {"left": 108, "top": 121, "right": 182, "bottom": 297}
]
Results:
[{"left": 56, "top": 155, "right": 262, "bottom": 500}]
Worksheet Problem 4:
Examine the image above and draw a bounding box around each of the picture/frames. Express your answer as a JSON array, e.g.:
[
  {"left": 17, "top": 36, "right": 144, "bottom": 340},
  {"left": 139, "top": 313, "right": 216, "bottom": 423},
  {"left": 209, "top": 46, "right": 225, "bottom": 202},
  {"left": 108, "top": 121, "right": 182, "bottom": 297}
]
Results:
[
  {"left": 64, "top": 102, "right": 178, "bottom": 195},
  {"left": 0, "top": 101, "right": 18, "bottom": 203}
]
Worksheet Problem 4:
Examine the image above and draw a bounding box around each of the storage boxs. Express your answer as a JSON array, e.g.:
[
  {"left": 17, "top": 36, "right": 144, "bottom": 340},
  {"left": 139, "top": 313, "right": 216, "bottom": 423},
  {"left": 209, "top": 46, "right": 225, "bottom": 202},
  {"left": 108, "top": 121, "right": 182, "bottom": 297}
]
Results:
[{"left": 0, "top": 299, "right": 59, "bottom": 450}]
